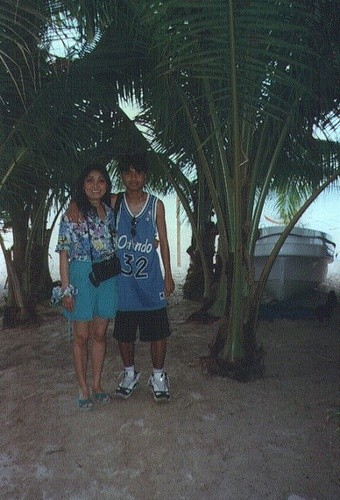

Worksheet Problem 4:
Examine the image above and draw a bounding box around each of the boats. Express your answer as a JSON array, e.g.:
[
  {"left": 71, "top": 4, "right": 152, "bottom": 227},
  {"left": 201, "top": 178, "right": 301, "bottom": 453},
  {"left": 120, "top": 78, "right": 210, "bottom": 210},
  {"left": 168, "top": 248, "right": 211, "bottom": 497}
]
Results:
[{"left": 250, "top": 225, "right": 337, "bottom": 302}]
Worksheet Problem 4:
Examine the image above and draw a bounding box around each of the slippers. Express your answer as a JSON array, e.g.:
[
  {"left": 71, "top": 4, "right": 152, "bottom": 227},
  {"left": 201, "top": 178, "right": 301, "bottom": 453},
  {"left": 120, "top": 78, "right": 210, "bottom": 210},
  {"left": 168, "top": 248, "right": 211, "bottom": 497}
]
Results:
[
  {"left": 96, "top": 392, "right": 113, "bottom": 404},
  {"left": 78, "top": 397, "right": 93, "bottom": 410}
]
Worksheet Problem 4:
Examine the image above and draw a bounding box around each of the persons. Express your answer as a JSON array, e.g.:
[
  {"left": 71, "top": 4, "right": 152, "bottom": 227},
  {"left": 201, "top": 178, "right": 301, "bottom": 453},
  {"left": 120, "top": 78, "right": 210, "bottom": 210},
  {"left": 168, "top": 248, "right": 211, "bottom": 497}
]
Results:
[
  {"left": 52, "top": 162, "right": 119, "bottom": 410},
  {"left": 67, "top": 153, "right": 175, "bottom": 401}
]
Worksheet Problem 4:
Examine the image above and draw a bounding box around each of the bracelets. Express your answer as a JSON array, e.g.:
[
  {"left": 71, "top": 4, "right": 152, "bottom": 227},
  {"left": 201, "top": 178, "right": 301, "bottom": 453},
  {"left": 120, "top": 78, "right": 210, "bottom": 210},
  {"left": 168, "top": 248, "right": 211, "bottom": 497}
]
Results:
[{"left": 52, "top": 284, "right": 79, "bottom": 307}]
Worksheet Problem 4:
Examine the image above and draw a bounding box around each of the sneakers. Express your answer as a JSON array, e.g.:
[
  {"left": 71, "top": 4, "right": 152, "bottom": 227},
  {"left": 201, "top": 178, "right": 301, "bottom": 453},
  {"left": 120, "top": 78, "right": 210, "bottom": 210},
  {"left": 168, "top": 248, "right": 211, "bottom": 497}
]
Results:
[
  {"left": 116, "top": 370, "right": 140, "bottom": 398},
  {"left": 152, "top": 371, "right": 170, "bottom": 403}
]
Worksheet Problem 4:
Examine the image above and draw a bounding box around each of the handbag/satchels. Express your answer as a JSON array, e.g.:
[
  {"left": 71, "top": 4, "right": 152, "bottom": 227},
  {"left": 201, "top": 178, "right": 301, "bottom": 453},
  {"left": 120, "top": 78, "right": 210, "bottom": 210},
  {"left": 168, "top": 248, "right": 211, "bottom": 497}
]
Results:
[{"left": 89, "top": 256, "right": 121, "bottom": 287}]
